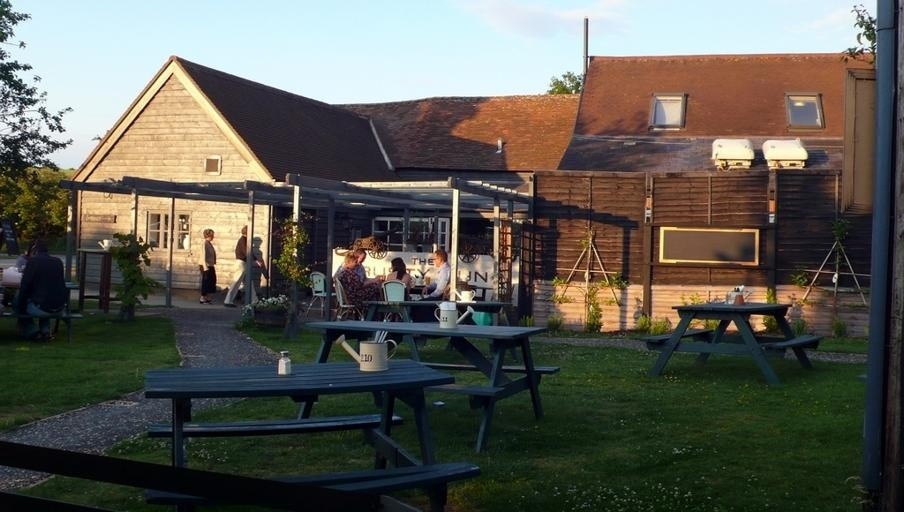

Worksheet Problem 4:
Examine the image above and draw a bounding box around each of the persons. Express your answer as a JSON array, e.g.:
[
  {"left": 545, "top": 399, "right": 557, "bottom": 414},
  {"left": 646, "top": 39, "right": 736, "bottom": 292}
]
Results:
[
  {"left": 354, "top": 249, "right": 385, "bottom": 287},
  {"left": 14, "top": 240, "right": 39, "bottom": 338},
  {"left": 223, "top": 226, "right": 259, "bottom": 308},
  {"left": 417, "top": 249, "right": 451, "bottom": 300},
  {"left": 382, "top": 257, "right": 412, "bottom": 321},
  {"left": 337, "top": 252, "right": 381, "bottom": 321},
  {"left": 11, "top": 244, "right": 67, "bottom": 345},
  {"left": 197, "top": 229, "right": 216, "bottom": 305}
]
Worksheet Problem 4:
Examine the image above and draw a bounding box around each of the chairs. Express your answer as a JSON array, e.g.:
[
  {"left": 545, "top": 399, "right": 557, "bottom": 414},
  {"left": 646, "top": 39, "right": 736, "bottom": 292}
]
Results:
[
  {"left": 306, "top": 272, "right": 357, "bottom": 321},
  {"left": 382, "top": 280, "right": 407, "bottom": 301}
]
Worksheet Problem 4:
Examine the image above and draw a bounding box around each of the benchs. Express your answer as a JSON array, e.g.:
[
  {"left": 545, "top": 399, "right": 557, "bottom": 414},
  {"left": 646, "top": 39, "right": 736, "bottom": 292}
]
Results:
[
  {"left": 634, "top": 328, "right": 825, "bottom": 386},
  {"left": 1, "top": 312, "right": 84, "bottom": 345}
]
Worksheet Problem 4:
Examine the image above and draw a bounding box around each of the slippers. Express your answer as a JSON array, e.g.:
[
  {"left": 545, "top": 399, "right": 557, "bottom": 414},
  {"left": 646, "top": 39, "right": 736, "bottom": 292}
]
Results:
[{"left": 200, "top": 297, "right": 213, "bottom": 305}]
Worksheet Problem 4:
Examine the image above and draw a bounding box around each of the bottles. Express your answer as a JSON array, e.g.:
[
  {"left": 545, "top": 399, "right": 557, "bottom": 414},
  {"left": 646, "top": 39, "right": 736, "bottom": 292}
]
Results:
[{"left": 277, "top": 351, "right": 291, "bottom": 375}]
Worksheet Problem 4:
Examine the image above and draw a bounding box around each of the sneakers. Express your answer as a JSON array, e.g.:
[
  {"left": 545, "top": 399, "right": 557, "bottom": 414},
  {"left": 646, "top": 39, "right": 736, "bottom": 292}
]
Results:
[{"left": 224, "top": 302, "right": 236, "bottom": 308}]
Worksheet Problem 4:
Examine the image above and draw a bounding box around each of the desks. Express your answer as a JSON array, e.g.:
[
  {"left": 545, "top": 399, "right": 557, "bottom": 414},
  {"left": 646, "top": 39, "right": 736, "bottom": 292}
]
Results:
[
  {"left": 652, "top": 301, "right": 814, "bottom": 386},
  {"left": 296, "top": 301, "right": 545, "bottom": 455},
  {"left": 1, "top": 282, "right": 80, "bottom": 344}
]
